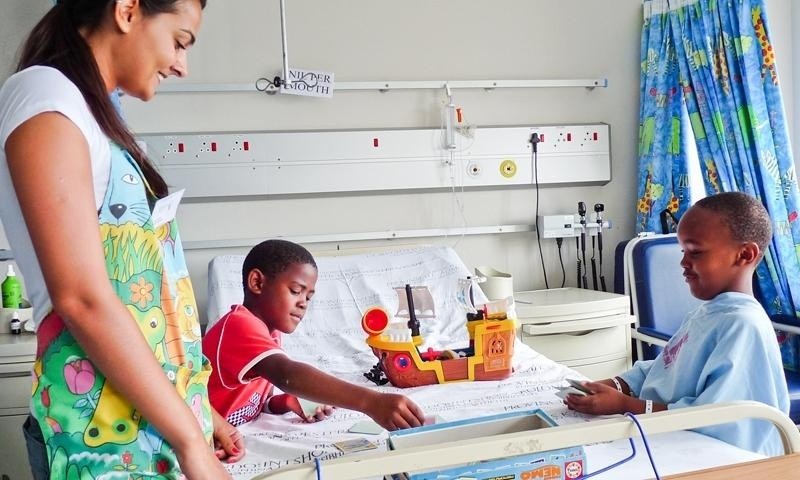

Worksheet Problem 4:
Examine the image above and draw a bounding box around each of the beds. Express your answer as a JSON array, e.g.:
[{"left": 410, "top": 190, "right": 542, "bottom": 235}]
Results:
[{"left": 204, "top": 244, "right": 800, "bottom": 480}]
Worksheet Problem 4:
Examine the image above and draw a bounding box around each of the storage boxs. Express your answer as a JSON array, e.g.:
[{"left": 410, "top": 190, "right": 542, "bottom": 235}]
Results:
[{"left": 384, "top": 406, "right": 590, "bottom": 480}]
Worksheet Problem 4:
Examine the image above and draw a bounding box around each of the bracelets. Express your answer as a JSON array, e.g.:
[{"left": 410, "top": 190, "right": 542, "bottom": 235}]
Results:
[
  {"left": 611, "top": 377, "right": 623, "bottom": 392},
  {"left": 645, "top": 400, "right": 653, "bottom": 414}
]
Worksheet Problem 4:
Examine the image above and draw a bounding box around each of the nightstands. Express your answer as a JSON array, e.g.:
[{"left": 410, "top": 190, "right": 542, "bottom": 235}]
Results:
[
  {"left": 514, "top": 286, "right": 632, "bottom": 383},
  {"left": 0, "top": 327, "right": 49, "bottom": 480}
]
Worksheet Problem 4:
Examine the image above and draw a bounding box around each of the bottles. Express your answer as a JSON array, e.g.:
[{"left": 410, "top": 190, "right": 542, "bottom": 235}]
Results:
[
  {"left": 11, "top": 311, "right": 21, "bottom": 334},
  {"left": 2, "top": 264, "right": 22, "bottom": 308}
]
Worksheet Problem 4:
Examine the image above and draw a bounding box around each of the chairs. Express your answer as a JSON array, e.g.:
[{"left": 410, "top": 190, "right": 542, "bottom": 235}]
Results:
[{"left": 614, "top": 231, "right": 800, "bottom": 428}]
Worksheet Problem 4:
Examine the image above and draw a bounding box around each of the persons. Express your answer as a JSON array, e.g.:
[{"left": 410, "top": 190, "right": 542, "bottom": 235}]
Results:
[
  {"left": 0, "top": 0, "right": 247, "bottom": 480},
  {"left": 563, "top": 191, "right": 790, "bottom": 453},
  {"left": 202, "top": 240, "right": 425, "bottom": 448}
]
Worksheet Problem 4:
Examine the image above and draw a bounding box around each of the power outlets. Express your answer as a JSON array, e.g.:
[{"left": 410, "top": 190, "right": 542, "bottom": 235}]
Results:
[
  {"left": 158, "top": 136, "right": 249, "bottom": 165},
  {"left": 532, "top": 123, "right": 609, "bottom": 154}
]
